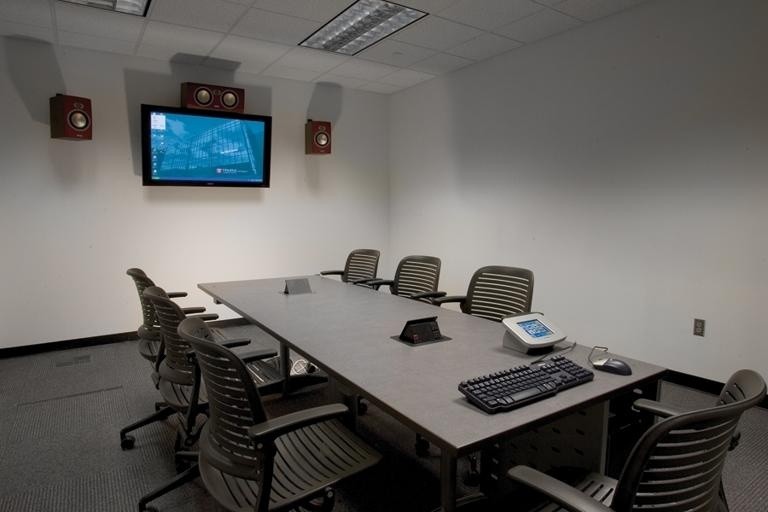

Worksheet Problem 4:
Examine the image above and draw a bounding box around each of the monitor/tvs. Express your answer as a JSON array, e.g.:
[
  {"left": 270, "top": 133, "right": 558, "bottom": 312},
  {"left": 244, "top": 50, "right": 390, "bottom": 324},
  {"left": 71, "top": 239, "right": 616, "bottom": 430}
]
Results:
[{"left": 140, "top": 102, "right": 272, "bottom": 187}]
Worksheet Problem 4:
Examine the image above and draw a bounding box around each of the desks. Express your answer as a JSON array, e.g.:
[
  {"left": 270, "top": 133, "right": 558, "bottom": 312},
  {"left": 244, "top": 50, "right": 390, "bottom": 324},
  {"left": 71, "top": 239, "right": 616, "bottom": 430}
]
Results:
[{"left": 191, "top": 274, "right": 669, "bottom": 512}]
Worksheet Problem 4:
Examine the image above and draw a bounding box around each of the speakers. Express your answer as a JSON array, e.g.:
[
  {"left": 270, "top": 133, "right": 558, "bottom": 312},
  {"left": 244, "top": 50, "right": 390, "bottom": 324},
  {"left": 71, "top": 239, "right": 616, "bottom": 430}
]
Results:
[
  {"left": 305, "top": 120, "right": 331, "bottom": 154},
  {"left": 181, "top": 82, "right": 245, "bottom": 114},
  {"left": 49, "top": 95, "right": 93, "bottom": 141}
]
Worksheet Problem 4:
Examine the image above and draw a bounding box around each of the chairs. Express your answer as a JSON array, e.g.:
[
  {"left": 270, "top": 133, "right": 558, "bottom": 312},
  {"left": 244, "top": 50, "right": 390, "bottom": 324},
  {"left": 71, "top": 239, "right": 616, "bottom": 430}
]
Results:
[
  {"left": 114, "top": 247, "right": 545, "bottom": 511},
  {"left": 506, "top": 368, "right": 763, "bottom": 512}
]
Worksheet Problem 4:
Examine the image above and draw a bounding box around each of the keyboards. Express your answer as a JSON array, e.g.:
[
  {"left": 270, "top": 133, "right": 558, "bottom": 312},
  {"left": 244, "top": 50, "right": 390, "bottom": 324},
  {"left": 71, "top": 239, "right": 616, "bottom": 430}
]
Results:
[{"left": 458, "top": 354, "right": 594, "bottom": 413}]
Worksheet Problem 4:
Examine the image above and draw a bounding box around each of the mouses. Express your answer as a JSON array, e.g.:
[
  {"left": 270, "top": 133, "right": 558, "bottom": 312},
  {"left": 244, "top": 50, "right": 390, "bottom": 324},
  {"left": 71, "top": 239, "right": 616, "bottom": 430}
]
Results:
[{"left": 593, "top": 357, "right": 632, "bottom": 376}]
